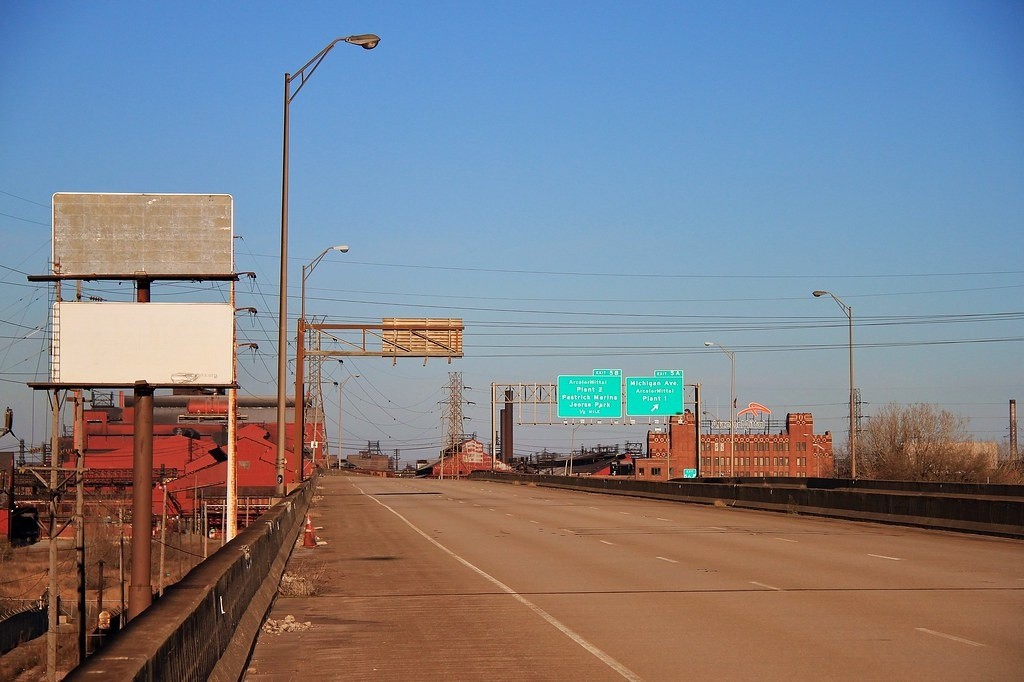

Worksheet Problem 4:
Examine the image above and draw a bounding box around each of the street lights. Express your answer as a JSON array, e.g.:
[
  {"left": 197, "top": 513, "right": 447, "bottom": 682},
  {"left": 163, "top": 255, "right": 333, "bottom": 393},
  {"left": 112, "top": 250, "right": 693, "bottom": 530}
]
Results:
[
  {"left": 339, "top": 374, "right": 360, "bottom": 469},
  {"left": 704, "top": 342, "right": 735, "bottom": 477},
  {"left": 703, "top": 411, "right": 721, "bottom": 477},
  {"left": 813, "top": 291, "right": 856, "bottom": 478},
  {"left": 570, "top": 424, "right": 587, "bottom": 476},
  {"left": 277, "top": 34, "right": 382, "bottom": 495},
  {"left": 804, "top": 434, "right": 820, "bottom": 477},
  {"left": 302, "top": 246, "right": 350, "bottom": 319}
]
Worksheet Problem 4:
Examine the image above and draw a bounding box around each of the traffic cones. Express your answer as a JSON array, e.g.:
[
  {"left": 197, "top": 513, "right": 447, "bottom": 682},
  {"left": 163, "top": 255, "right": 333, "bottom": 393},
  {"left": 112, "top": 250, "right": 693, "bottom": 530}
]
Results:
[{"left": 302, "top": 512, "right": 319, "bottom": 548}]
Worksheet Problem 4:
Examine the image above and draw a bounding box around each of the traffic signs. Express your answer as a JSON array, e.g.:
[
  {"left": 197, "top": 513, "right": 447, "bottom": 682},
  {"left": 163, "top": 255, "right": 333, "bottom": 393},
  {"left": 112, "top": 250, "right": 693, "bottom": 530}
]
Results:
[
  {"left": 556, "top": 368, "right": 623, "bottom": 421},
  {"left": 625, "top": 371, "right": 685, "bottom": 417}
]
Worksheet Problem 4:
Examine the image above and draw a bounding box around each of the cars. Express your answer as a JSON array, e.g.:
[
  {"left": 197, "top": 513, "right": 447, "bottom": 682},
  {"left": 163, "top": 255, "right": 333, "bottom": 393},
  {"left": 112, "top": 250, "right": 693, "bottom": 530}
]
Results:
[{"left": 331, "top": 459, "right": 360, "bottom": 470}]
